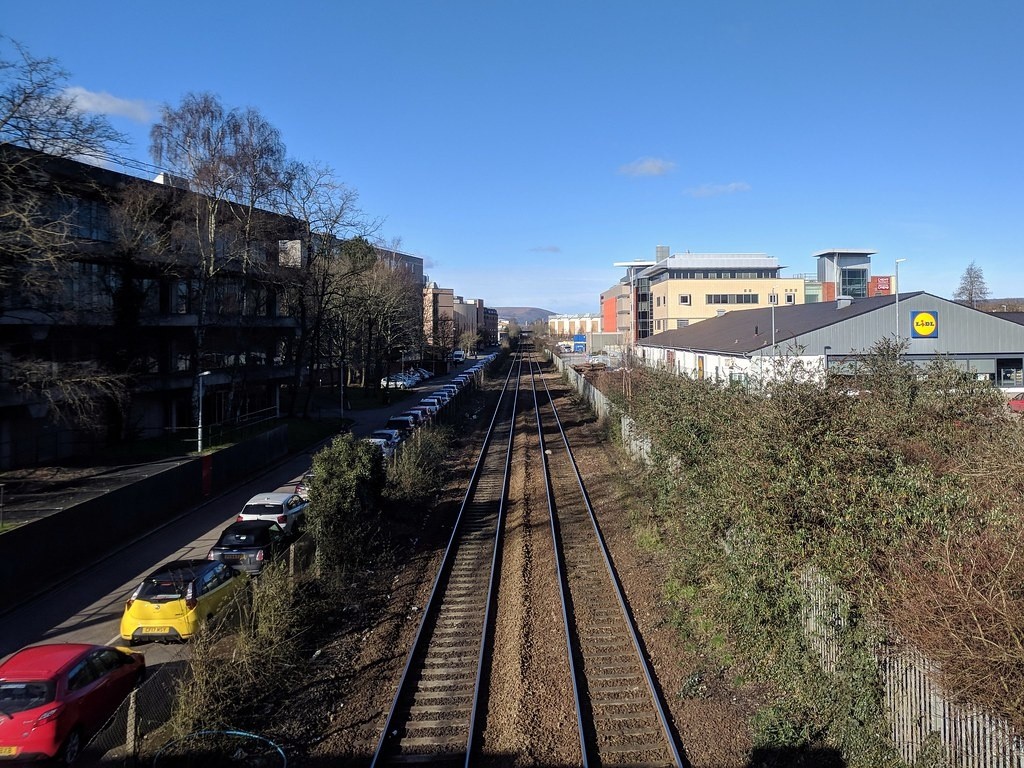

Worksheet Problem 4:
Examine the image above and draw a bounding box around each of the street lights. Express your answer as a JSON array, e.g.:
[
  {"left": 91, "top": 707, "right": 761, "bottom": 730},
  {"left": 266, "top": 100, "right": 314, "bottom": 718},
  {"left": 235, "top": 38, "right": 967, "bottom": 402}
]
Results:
[
  {"left": 198, "top": 371, "right": 210, "bottom": 453},
  {"left": 895, "top": 257, "right": 907, "bottom": 364},
  {"left": 771, "top": 285, "right": 781, "bottom": 384}
]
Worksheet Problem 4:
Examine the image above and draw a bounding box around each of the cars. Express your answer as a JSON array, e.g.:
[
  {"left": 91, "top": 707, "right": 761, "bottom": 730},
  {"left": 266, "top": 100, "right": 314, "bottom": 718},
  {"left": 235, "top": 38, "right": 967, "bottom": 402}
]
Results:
[
  {"left": 558, "top": 345, "right": 633, "bottom": 373},
  {"left": 371, "top": 430, "right": 401, "bottom": 447},
  {"left": 361, "top": 439, "right": 392, "bottom": 460},
  {"left": 381, "top": 350, "right": 501, "bottom": 427},
  {"left": 0, "top": 642, "right": 147, "bottom": 767},
  {"left": 385, "top": 416, "right": 416, "bottom": 439},
  {"left": 120, "top": 559, "right": 251, "bottom": 646},
  {"left": 237, "top": 493, "right": 311, "bottom": 537},
  {"left": 294, "top": 472, "right": 317, "bottom": 502},
  {"left": 205, "top": 519, "right": 287, "bottom": 580}
]
